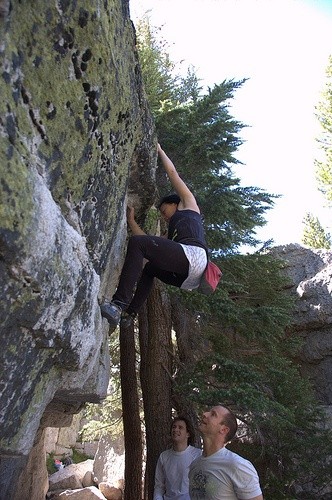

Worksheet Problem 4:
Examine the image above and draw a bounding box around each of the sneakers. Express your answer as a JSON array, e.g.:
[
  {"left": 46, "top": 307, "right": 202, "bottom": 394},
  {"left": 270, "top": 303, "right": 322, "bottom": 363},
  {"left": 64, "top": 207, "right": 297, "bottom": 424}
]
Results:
[
  {"left": 100, "top": 296, "right": 120, "bottom": 325},
  {"left": 120, "top": 312, "right": 133, "bottom": 328}
]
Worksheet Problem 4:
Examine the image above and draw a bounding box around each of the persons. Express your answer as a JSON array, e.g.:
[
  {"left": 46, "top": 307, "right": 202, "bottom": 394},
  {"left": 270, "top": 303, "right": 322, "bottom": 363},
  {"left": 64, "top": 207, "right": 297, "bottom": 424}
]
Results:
[
  {"left": 100, "top": 143, "right": 208, "bottom": 327},
  {"left": 154, "top": 418, "right": 202, "bottom": 500},
  {"left": 189, "top": 404, "right": 263, "bottom": 500}
]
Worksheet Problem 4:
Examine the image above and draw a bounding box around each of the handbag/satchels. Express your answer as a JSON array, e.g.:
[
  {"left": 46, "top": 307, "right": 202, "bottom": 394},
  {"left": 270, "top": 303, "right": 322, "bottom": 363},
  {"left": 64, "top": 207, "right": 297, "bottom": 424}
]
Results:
[{"left": 200, "top": 262, "right": 223, "bottom": 294}]
